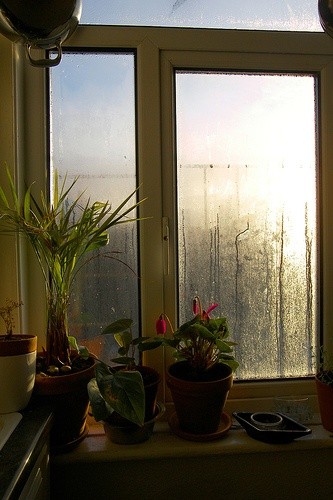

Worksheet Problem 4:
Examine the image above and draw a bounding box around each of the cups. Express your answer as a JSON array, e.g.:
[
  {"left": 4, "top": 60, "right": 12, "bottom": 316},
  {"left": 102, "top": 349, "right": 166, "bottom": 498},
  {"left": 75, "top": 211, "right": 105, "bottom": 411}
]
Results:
[
  {"left": 250, "top": 411, "right": 283, "bottom": 431},
  {"left": 273, "top": 395, "right": 311, "bottom": 426}
]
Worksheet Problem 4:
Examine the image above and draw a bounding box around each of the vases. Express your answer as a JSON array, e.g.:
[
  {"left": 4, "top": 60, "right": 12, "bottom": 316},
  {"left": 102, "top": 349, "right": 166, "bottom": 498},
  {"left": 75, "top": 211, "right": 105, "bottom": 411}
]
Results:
[{"left": 163, "top": 358, "right": 235, "bottom": 442}]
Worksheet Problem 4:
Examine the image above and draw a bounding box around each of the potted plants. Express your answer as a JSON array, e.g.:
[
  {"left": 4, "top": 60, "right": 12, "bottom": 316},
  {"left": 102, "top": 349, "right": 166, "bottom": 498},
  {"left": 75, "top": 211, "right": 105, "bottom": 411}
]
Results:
[
  {"left": 93, "top": 314, "right": 167, "bottom": 445},
  {"left": 0, "top": 296, "right": 39, "bottom": 415},
  {"left": 5, "top": 159, "right": 156, "bottom": 454},
  {"left": 310, "top": 340, "right": 333, "bottom": 432}
]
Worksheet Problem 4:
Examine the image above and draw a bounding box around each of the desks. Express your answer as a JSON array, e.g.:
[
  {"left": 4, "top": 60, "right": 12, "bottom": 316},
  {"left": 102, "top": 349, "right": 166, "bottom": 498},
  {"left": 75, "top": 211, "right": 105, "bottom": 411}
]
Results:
[{"left": 48, "top": 422, "right": 333, "bottom": 500}]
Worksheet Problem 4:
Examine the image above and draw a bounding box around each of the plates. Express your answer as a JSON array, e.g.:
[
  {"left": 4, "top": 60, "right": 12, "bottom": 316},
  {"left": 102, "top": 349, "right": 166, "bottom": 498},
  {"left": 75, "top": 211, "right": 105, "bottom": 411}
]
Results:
[{"left": 233, "top": 411, "right": 312, "bottom": 439}]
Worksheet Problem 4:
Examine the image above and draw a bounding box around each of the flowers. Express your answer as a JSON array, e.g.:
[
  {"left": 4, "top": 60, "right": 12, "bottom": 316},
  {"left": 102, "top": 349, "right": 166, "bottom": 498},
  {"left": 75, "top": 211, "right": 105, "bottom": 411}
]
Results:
[{"left": 153, "top": 295, "right": 241, "bottom": 374}]
